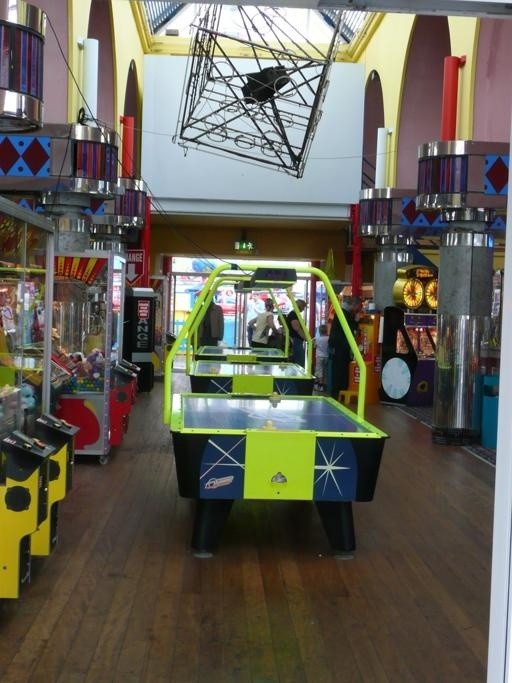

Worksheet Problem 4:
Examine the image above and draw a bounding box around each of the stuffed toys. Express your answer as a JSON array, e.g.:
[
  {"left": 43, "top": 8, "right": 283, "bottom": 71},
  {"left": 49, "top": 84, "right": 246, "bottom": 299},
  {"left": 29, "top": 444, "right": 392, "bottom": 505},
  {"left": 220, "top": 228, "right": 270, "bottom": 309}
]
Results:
[
  {"left": 86, "top": 349, "right": 106, "bottom": 378},
  {"left": 20, "top": 382, "right": 37, "bottom": 410},
  {"left": 70, "top": 351, "right": 88, "bottom": 376}
]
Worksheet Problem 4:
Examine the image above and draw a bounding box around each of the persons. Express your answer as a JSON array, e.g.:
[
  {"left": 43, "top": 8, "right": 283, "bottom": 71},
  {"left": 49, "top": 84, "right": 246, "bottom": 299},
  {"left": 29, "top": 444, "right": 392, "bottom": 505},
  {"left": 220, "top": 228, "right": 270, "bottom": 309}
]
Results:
[
  {"left": 191, "top": 293, "right": 224, "bottom": 347},
  {"left": 286, "top": 297, "right": 310, "bottom": 368},
  {"left": 248, "top": 298, "right": 280, "bottom": 346},
  {"left": 327, "top": 295, "right": 365, "bottom": 399},
  {"left": 311, "top": 324, "right": 330, "bottom": 391},
  {"left": 274, "top": 313, "right": 295, "bottom": 344}
]
